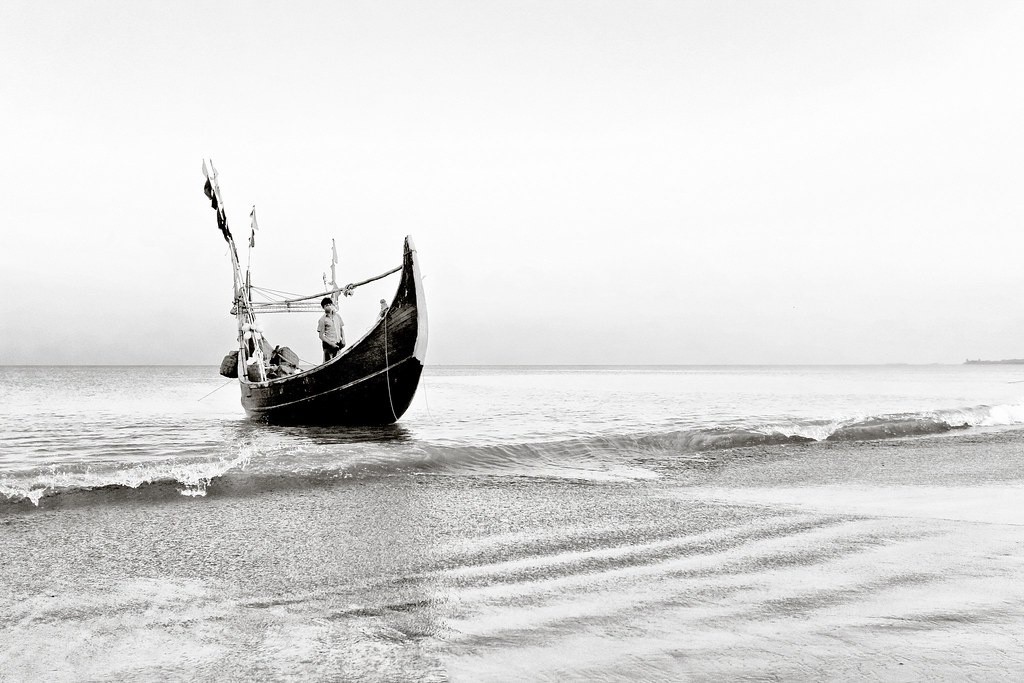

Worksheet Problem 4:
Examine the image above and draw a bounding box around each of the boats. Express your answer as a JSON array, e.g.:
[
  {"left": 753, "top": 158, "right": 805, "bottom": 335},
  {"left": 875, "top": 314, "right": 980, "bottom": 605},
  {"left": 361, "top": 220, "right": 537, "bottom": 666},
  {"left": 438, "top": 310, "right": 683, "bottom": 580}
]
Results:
[{"left": 201, "top": 155, "right": 428, "bottom": 427}]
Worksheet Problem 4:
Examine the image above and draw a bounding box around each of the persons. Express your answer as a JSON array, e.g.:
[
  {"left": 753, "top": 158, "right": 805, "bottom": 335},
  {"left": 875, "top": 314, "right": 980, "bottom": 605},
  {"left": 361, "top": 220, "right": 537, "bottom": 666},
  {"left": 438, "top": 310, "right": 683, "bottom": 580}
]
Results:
[{"left": 318, "top": 298, "right": 345, "bottom": 362}]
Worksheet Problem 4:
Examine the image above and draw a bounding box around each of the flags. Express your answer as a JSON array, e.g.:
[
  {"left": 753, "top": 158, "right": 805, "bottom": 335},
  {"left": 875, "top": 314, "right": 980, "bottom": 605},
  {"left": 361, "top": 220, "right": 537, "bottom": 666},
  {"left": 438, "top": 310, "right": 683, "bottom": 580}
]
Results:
[
  {"left": 202, "top": 162, "right": 233, "bottom": 242},
  {"left": 249, "top": 231, "right": 254, "bottom": 248},
  {"left": 333, "top": 244, "right": 338, "bottom": 262},
  {"left": 250, "top": 210, "right": 259, "bottom": 229}
]
